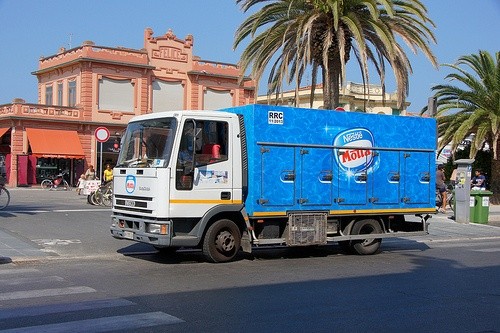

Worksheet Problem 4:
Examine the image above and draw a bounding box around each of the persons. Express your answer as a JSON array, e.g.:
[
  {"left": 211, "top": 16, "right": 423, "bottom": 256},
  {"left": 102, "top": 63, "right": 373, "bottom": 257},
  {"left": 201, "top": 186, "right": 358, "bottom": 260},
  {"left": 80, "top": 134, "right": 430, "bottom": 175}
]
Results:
[
  {"left": 84, "top": 165, "right": 99, "bottom": 180},
  {"left": 436, "top": 164, "right": 448, "bottom": 214},
  {"left": 450, "top": 169, "right": 457, "bottom": 186},
  {"left": 471, "top": 169, "right": 485, "bottom": 190},
  {"left": 76, "top": 174, "right": 85, "bottom": 195},
  {"left": 104, "top": 164, "right": 114, "bottom": 199}
]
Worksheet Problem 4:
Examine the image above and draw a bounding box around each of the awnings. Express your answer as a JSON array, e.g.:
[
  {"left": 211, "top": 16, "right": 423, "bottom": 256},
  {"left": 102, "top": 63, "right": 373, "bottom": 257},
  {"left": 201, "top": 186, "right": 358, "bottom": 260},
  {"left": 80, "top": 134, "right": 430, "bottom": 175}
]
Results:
[
  {"left": 0, "top": 127, "right": 10, "bottom": 137},
  {"left": 26, "top": 128, "right": 85, "bottom": 159}
]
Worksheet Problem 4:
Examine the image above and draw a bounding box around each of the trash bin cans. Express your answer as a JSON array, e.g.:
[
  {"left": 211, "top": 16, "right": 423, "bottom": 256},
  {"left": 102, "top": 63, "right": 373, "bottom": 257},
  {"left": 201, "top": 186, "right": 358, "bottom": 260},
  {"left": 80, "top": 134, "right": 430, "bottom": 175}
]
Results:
[
  {"left": 472, "top": 189, "right": 494, "bottom": 224},
  {"left": 451, "top": 190, "right": 475, "bottom": 223}
]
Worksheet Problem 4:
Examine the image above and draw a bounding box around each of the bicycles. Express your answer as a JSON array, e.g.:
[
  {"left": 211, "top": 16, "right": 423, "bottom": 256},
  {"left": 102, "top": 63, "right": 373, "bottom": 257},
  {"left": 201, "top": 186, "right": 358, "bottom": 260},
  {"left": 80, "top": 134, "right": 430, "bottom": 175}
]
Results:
[
  {"left": 88, "top": 179, "right": 112, "bottom": 206},
  {"left": 436, "top": 182, "right": 455, "bottom": 211},
  {"left": 41, "top": 173, "right": 70, "bottom": 191},
  {"left": 0, "top": 177, "right": 10, "bottom": 210}
]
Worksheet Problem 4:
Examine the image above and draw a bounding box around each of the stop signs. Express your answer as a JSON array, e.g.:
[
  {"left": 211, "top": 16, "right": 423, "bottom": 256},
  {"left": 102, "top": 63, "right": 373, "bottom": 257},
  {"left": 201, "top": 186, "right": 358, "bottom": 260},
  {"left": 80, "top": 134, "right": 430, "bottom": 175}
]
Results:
[{"left": 94, "top": 127, "right": 110, "bottom": 142}]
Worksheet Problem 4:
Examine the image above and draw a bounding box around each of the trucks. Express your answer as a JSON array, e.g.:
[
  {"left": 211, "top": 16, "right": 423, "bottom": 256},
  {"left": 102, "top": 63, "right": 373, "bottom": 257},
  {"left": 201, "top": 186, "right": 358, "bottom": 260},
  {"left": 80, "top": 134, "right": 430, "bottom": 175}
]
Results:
[{"left": 110, "top": 104, "right": 437, "bottom": 262}]
